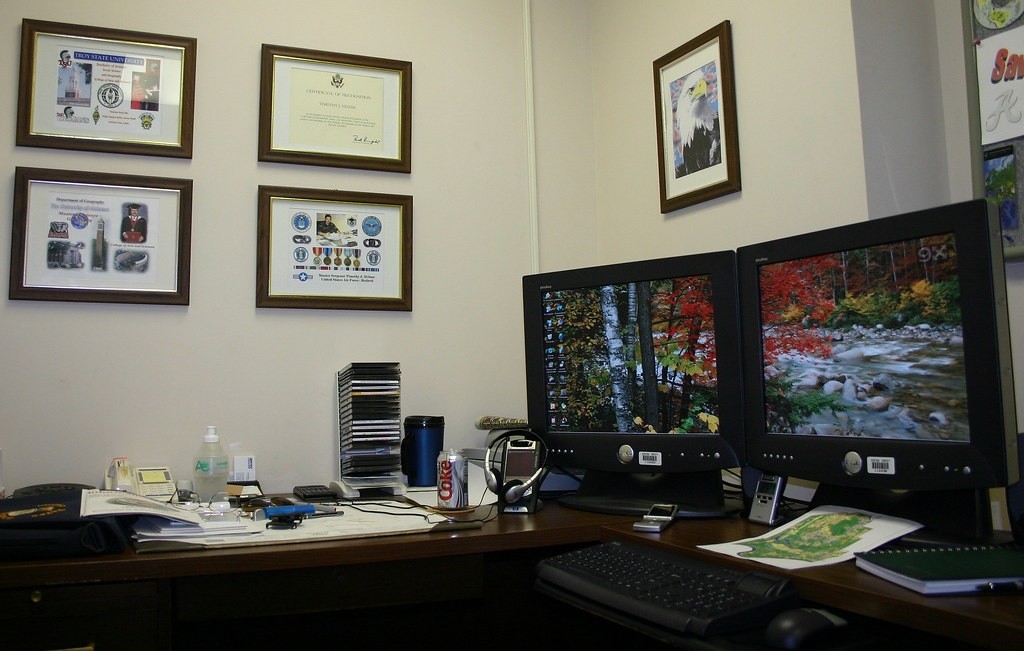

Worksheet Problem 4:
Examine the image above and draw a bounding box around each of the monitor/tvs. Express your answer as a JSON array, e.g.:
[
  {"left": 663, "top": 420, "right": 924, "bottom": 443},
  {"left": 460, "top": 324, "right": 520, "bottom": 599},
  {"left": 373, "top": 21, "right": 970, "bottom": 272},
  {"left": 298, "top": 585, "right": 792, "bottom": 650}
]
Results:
[
  {"left": 522, "top": 250, "right": 745, "bottom": 518},
  {"left": 736, "top": 199, "right": 1019, "bottom": 548}
]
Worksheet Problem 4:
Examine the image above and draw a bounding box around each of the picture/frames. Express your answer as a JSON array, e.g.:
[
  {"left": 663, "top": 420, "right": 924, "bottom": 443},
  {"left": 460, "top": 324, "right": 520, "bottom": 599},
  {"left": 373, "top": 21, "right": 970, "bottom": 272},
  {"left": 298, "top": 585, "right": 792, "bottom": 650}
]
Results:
[
  {"left": 653, "top": 20, "right": 742, "bottom": 214},
  {"left": 16, "top": 18, "right": 197, "bottom": 159},
  {"left": 256, "top": 184, "right": 413, "bottom": 311},
  {"left": 258, "top": 44, "right": 413, "bottom": 173},
  {"left": 9, "top": 167, "right": 193, "bottom": 305}
]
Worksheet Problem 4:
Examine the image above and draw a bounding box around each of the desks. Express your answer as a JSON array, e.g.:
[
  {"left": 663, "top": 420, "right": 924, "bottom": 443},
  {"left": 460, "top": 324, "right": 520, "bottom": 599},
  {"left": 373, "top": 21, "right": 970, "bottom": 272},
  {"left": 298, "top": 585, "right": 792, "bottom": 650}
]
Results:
[{"left": 0, "top": 446, "right": 1024, "bottom": 651}]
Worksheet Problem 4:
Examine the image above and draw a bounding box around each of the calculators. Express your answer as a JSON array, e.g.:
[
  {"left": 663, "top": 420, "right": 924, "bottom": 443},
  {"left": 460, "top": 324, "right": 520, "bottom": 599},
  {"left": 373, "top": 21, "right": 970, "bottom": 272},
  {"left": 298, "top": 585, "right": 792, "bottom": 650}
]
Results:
[{"left": 293, "top": 485, "right": 331, "bottom": 503}]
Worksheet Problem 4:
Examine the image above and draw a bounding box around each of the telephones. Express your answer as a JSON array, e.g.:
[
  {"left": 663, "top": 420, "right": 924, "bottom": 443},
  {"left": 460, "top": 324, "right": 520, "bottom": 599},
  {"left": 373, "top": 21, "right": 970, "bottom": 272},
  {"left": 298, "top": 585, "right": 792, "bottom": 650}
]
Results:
[{"left": 104, "top": 457, "right": 179, "bottom": 503}]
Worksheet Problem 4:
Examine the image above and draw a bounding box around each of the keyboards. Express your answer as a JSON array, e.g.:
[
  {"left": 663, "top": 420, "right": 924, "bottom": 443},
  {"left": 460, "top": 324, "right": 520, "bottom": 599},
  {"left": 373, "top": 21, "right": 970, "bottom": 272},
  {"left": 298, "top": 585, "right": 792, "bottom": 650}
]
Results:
[{"left": 534, "top": 539, "right": 795, "bottom": 641}]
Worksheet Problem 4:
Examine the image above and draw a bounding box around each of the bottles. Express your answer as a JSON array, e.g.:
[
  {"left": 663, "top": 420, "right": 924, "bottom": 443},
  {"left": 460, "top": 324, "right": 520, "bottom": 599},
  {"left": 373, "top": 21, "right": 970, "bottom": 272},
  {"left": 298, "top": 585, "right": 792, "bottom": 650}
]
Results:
[{"left": 192, "top": 426, "right": 229, "bottom": 503}]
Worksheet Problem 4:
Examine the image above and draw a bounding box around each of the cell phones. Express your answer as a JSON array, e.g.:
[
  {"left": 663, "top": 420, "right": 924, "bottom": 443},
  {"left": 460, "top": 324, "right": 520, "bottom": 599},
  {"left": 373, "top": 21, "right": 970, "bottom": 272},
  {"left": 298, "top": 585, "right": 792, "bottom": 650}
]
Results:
[{"left": 633, "top": 503, "right": 678, "bottom": 533}]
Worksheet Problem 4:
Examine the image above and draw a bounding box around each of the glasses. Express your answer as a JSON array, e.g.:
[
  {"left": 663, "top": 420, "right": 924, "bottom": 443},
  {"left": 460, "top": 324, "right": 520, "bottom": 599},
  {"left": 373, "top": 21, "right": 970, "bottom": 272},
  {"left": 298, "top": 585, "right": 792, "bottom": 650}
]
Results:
[{"left": 166, "top": 488, "right": 239, "bottom": 513}]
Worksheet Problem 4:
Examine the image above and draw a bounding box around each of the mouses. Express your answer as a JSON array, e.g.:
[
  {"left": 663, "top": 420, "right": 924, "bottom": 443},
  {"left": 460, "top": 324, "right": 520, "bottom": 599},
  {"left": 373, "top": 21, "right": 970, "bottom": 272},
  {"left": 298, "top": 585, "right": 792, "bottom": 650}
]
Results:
[{"left": 766, "top": 607, "right": 847, "bottom": 651}]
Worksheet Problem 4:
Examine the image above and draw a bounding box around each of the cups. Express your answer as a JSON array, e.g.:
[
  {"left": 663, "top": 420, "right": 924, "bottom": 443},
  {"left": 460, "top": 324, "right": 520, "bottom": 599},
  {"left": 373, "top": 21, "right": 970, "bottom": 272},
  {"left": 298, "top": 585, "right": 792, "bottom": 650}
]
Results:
[{"left": 403, "top": 415, "right": 445, "bottom": 486}]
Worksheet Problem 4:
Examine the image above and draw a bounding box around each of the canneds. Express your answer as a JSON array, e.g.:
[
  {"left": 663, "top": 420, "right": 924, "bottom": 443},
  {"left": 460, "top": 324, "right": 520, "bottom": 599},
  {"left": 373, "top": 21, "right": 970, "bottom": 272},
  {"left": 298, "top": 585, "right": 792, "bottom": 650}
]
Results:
[{"left": 437, "top": 449, "right": 468, "bottom": 508}]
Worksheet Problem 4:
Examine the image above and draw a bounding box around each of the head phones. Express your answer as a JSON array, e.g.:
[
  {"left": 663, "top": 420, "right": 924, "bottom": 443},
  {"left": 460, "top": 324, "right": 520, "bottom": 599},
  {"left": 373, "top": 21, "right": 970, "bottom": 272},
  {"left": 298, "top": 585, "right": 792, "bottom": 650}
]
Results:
[{"left": 484, "top": 429, "right": 547, "bottom": 503}]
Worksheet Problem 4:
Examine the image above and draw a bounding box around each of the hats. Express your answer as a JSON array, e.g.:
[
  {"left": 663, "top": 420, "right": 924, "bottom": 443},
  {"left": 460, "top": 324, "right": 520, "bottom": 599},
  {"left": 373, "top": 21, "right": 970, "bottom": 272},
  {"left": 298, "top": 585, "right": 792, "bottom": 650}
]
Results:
[{"left": 127, "top": 204, "right": 142, "bottom": 209}]
